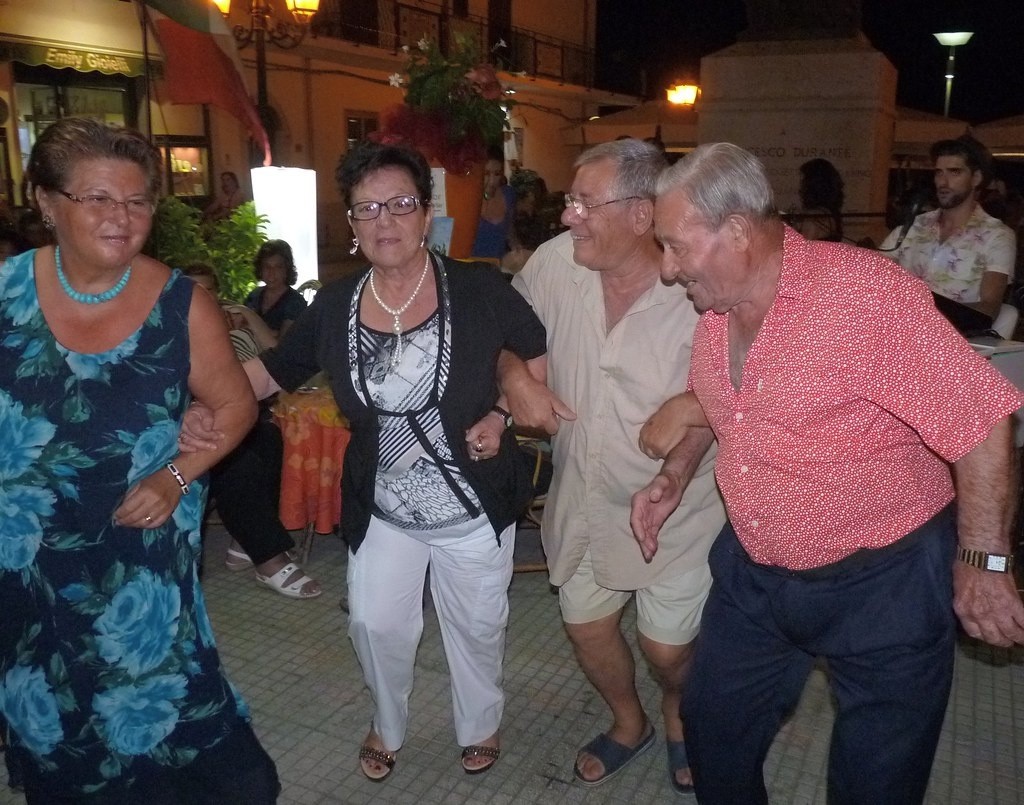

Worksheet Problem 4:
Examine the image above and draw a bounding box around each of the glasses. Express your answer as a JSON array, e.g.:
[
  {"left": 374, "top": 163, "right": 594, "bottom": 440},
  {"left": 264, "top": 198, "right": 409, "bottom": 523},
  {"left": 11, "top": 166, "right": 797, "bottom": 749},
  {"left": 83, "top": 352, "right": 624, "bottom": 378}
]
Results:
[
  {"left": 54, "top": 189, "right": 156, "bottom": 219},
  {"left": 565, "top": 193, "right": 643, "bottom": 221},
  {"left": 347, "top": 195, "right": 424, "bottom": 220}
]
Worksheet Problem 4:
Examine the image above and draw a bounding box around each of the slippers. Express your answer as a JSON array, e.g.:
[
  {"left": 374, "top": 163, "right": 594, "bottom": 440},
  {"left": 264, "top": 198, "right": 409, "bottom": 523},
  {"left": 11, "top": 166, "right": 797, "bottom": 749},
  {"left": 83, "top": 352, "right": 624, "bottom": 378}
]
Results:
[
  {"left": 667, "top": 737, "right": 696, "bottom": 796},
  {"left": 574, "top": 725, "right": 656, "bottom": 786}
]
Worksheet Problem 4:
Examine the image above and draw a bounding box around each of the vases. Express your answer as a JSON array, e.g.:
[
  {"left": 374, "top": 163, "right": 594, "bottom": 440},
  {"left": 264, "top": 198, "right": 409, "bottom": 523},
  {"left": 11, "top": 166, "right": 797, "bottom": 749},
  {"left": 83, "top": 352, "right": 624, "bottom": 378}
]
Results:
[{"left": 419, "top": 151, "right": 485, "bottom": 259}]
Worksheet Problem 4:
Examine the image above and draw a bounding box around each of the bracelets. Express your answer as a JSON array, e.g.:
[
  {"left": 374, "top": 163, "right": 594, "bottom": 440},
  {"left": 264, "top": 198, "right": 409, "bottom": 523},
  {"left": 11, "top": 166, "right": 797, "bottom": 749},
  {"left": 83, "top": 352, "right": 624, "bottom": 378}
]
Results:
[{"left": 167, "top": 463, "right": 190, "bottom": 496}]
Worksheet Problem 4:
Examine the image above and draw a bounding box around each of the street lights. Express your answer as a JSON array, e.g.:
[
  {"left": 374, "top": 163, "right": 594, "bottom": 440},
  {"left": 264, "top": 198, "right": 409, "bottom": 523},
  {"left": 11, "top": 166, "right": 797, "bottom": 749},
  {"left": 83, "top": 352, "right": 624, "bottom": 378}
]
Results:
[
  {"left": 932, "top": 32, "right": 974, "bottom": 117},
  {"left": 655, "top": 57, "right": 699, "bottom": 139},
  {"left": 213, "top": 0, "right": 319, "bottom": 128}
]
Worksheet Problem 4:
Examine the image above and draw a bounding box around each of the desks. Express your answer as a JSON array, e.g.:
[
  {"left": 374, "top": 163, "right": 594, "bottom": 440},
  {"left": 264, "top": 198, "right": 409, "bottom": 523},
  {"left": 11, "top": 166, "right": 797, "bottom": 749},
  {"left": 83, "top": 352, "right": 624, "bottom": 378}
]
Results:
[{"left": 273, "top": 381, "right": 349, "bottom": 567}]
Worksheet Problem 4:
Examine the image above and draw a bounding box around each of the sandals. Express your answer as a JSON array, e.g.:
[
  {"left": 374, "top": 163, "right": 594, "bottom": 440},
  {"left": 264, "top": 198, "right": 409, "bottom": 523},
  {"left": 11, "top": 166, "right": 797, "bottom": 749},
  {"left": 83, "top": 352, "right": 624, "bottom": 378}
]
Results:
[
  {"left": 255, "top": 562, "right": 323, "bottom": 599},
  {"left": 461, "top": 726, "right": 500, "bottom": 772},
  {"left": 225, "top": 548, "right": 256, "bottom": 573},
  {"left": 360, "top": 719, "right": 398, "bottom": 781}
]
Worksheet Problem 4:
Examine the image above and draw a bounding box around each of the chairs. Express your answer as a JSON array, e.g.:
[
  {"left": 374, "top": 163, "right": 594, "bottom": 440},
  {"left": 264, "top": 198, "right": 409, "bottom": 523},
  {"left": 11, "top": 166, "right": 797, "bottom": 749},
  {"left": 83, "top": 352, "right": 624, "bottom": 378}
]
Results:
[{"left": 513, "top": 428, "right": 547, "bottom": 571}]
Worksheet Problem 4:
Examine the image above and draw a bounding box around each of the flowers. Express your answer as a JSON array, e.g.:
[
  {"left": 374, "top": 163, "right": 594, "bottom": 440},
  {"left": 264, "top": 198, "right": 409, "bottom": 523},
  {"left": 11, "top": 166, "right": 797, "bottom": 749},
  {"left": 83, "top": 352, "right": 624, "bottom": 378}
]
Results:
[{"left": 387, "top": 28, "right": 515, "bottom": 140}]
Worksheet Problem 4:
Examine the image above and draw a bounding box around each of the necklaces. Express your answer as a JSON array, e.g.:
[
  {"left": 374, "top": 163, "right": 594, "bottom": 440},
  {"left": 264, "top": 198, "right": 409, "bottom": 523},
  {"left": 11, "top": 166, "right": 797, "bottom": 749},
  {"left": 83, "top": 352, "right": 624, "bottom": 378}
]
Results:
[
  {"left": 370, "top": 252, "right": 429, "bottom": 367},
  {"left": 55, "top": 245, "right": 132, "bottom": 304}
]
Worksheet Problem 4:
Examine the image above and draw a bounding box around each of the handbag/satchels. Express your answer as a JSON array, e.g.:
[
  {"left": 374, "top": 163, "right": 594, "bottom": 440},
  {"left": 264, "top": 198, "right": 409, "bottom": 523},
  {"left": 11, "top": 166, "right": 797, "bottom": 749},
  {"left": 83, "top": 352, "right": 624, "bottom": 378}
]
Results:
[{"left": 226, "top": 310, "right": 262, "bottom": 367}]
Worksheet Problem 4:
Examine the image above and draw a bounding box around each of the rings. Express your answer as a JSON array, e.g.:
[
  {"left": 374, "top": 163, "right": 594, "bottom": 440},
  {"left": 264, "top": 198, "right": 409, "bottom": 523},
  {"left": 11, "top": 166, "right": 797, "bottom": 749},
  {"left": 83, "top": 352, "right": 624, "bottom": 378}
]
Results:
[
  {"left": 145, "top": 515, "right": 153, "bottom": 524},
  {"left": 178, "top": 432, "right": 186, "bottom": 443},
  {"left": 475, "top": 456, "right": 479, "bottom": 462},
  {"left": 477, "top": 442, "right": 481, "bottom": 451}
]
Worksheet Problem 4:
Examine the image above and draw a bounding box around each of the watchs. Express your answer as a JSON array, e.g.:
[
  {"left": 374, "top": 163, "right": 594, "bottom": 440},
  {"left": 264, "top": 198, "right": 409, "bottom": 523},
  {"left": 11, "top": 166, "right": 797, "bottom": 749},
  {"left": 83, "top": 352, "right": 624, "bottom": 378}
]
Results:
[
  {"left": 956, "top": 546, "right": 1014, "bottom": 574},
  {"left": 492, "top": 406, "right": 512, "bottom": 430}
]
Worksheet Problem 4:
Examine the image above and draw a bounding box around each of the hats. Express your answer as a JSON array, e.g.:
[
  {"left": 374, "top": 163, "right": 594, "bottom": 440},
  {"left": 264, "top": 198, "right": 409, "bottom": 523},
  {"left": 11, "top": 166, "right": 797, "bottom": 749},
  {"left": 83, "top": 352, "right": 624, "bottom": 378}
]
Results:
[{"left": 929, "top": 135, "right": 994, "bottom": 192}]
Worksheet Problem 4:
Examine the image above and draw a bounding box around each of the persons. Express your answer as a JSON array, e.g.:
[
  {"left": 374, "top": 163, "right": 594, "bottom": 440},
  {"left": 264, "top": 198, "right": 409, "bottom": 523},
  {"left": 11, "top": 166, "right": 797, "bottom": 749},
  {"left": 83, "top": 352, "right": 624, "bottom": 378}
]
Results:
[
  {"left": 471, "top": 148, "right": 524, "bottom": 260},
  {"left": 639, "top": 142, "right": 1024, "bottom": 805},
  {"left": 877, "top": 134, "right": 1017, "bottom": 323},
  {"left": 796, "top": 158, "right": 845, "bottom": 243},
  {"left": 179, "top": 239, "right": 327, "bottom": 600},
  {"left": 0, "top": 117, "right": 282, "bottom": 805},
  {"left": 495, "top": 136, "right": 727, "bottom": 797},
  {"left": 178, "top": 141, "right": 548, "bottom": 783}
]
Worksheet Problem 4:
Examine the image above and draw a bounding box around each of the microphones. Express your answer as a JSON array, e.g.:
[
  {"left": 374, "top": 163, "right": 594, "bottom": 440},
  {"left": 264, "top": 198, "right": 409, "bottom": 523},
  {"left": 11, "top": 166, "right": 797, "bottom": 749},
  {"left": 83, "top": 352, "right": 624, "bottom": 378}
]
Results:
[{"left": 896, "top": 191, "right": 925, "bottom": 245}]
